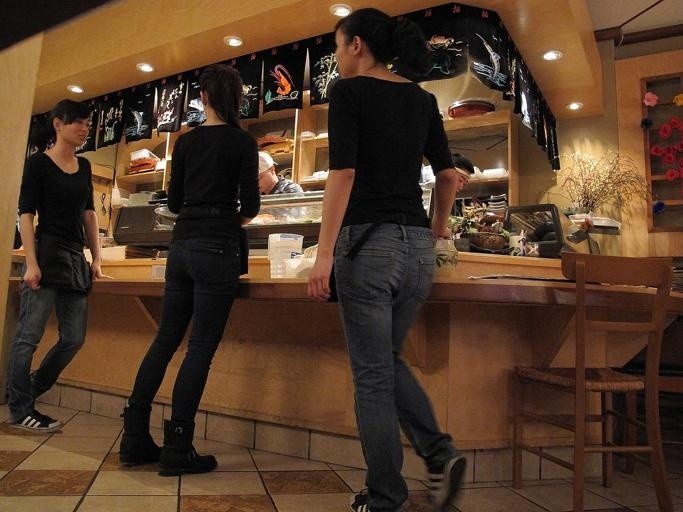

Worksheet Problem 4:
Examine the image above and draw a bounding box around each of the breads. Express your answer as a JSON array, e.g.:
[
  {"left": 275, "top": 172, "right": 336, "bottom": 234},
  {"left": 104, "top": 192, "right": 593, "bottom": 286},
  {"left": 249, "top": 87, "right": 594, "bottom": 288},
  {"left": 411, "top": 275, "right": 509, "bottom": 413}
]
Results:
[{"left": 251, "top": 214, "right": 274, "bottom": 223}]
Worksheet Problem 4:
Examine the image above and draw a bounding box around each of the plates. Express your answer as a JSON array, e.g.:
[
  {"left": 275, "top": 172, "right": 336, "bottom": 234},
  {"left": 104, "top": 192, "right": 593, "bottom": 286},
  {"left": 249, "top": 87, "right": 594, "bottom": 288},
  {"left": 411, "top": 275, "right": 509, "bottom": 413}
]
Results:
[
  {"left": 470, "top": 241, "right": 515, "bottom": 255},
  {"left": 485, "top": 193, "right": 508, "bottom": 212}
]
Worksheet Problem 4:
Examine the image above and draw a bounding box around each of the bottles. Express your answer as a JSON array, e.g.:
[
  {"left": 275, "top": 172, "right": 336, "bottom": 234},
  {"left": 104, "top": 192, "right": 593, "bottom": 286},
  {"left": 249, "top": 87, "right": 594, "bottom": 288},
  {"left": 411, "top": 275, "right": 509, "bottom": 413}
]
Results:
[{"left": 155, "top": 157, "right": 164, "bottom": 171}]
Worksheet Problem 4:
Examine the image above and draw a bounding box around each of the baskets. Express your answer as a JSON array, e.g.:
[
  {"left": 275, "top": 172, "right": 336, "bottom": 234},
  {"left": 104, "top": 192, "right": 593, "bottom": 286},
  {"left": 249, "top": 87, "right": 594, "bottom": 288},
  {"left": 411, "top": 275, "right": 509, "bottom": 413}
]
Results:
[{"left": 460, "top": 208, "right": 504, "bottom": 250}]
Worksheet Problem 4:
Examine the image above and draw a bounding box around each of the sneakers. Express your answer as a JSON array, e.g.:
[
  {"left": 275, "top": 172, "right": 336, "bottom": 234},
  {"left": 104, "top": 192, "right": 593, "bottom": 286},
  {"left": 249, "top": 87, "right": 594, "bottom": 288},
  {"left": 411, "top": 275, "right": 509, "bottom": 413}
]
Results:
[
  {"left": 427, "top": 453, "right": 465, "bottom": 509},
  {"left": 349, "top": 493, "right": 410, "bottom": 512},
  {"left": 9, "top": 409, "right": 62, "bottom": 434}
]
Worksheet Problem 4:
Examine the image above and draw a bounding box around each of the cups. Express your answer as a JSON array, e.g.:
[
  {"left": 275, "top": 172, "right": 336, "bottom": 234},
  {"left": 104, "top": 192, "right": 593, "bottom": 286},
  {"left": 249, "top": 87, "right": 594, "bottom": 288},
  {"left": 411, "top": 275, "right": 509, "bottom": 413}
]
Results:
[
  {"left": 452, "top": 239, "right": 471, "bottom": 251},
  {"left": 267, "top": 233, "right": 304, "bottom": 278}
]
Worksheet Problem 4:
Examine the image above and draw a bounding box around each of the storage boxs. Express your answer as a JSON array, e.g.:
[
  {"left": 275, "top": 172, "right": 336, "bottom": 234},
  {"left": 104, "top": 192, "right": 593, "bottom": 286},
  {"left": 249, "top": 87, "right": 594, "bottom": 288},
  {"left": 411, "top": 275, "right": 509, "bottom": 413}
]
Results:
[{"left": 91, "top": 162, "right": 115, "bottom": 231}]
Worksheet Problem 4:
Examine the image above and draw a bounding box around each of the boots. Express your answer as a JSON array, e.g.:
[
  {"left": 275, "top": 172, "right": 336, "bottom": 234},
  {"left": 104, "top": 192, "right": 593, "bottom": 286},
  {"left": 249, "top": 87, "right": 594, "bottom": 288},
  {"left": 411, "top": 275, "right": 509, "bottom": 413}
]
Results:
[
  {"left": 159, "top": 420, "right": 216, "bottom": 477},
  {"left": 119, "top": 407, "right": 163, "bottom": 467}
]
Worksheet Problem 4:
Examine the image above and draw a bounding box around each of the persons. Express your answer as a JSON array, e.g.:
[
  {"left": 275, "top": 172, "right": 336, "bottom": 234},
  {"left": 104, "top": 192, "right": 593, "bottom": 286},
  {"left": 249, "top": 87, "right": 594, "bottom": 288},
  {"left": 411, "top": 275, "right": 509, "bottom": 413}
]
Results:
[
  {"left": 305, "top": 7, "right": 467, "bottom": 510},
  {"left": 446, "top": 153, "right": 474, "bottom": 193},
  {"left": 7, "top": 99, "right": 102, "bottom": 432},
  {"left": 256, "top": 151, "right": 305, "bottom": 218},
  {"left": 119, "top": 64, "right": 259, "bottom": 475}
]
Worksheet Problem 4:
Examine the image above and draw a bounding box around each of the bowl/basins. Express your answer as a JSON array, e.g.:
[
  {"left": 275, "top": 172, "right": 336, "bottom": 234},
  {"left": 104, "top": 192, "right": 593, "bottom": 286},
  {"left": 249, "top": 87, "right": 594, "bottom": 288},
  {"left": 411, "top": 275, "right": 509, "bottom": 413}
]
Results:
[
  {"left": 98, "top": 244, "right": 127, "bottom": 262},
  {"left": 446, "top": 99, "right": 496, "bottom": 118},
  {"left": 482, "top": 168, "right": 506, "bottom": 179},
  {"left": 459, "top": 233, "right": 505, "bottom": 250},
  {"left": 282, "top": 258, "right": 315, "bottom": 280}
]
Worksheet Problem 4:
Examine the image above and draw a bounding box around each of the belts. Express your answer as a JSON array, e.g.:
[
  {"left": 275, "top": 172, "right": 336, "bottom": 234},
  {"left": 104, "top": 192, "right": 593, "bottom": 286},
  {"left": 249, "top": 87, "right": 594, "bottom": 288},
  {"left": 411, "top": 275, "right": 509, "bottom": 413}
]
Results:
[{"left": 341, "top": 212, "right": 431, "bottom": 228}]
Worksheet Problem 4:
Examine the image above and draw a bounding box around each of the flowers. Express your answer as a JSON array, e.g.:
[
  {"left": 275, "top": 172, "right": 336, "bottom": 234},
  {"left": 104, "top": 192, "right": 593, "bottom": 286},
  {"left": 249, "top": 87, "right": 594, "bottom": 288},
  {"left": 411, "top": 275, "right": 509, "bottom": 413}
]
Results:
[{"left": 639, "top": 91, "right": 682, "bottom": 213}]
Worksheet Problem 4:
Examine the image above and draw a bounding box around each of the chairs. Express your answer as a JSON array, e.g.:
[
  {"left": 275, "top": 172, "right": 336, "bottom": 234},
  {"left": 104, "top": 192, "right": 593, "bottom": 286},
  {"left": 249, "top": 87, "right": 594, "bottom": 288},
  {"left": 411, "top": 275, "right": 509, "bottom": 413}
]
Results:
[{"left": 510, "top": 253, "right": 676, "bottom": 511}]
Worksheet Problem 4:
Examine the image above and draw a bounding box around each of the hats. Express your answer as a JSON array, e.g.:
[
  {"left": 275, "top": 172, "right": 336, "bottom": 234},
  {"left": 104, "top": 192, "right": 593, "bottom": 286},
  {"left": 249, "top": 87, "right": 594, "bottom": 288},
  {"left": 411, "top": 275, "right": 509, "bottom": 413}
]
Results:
[{"left": 257, "top": 151, "right": 277, "bottom": 173}]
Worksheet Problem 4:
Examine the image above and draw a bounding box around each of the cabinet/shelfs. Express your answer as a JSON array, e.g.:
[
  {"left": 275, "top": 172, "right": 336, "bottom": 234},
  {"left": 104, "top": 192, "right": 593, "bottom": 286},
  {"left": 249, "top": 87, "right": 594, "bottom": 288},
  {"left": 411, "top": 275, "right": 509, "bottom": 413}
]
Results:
[
  {"left": 106, "top": 90, "right": 557, "bottom": 242},
  {"left": 640, "top": 71, "right": 683, "bottom": 233}
]
